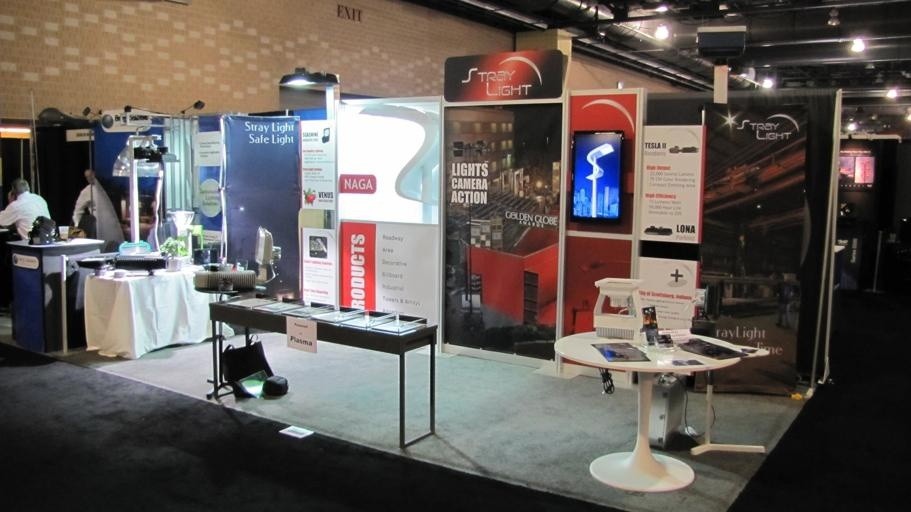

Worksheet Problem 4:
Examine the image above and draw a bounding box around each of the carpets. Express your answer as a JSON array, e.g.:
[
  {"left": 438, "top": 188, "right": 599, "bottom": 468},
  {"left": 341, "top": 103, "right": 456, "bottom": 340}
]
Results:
[{"left": 0, "top": 310, "right": 820, "bottom": 512}]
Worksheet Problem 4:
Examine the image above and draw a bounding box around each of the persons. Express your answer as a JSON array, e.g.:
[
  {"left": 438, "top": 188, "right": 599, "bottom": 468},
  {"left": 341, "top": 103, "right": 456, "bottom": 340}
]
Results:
[
  {"left": 0, "top": 178, "right": 52, "bottom": 241},
  {"left": 71, "top": 169, "right": 97, "bottom": 233}
]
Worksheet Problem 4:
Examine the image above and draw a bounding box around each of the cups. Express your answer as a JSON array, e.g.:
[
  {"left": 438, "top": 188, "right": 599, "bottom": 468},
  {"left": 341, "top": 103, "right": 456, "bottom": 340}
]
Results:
[{"left": 58, "top": 225, "right": 70, "bottom": 241}]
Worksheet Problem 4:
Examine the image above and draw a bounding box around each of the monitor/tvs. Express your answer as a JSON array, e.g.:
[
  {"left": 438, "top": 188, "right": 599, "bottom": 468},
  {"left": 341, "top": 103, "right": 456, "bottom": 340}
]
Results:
[{"left": 569, "top": 130, "right": 625, "bottom": 226}]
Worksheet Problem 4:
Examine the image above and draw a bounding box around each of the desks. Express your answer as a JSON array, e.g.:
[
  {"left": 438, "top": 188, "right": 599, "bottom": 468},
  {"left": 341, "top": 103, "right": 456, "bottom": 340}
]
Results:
[{"left": 554, "top": 330, "right": 771, "bottom": 493}]
